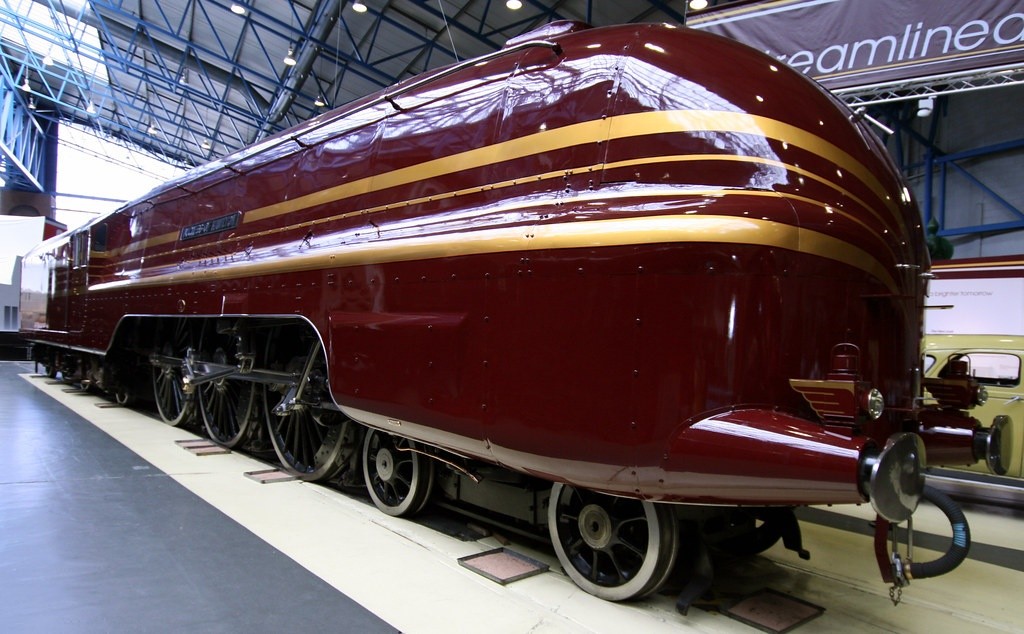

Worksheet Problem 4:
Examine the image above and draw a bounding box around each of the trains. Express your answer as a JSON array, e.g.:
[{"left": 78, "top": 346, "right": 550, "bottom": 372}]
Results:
[
  {"left": 923, "top": 333, "right": 1024, "bottom": 490},
  {"left": 14, "top": 24, "right": 1011, "bottom": 604}
]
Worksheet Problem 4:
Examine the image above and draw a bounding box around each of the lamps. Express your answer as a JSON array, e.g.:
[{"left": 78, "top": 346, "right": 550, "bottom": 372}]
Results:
[
  {"left": 27, "top": 93, "right": 37, "bottom": 109},
  {"left": 22, "top": 51, "right": 32, "bottom": 92},
  {"left": 202, "top": 136, "right": 210, "bottom": 148},
  {"left": 313, "top": 52, "right": 325, "bottom": 108},
  {"left": 148, "top": 121, "right": 158, "bottom": 135},
  {"left": 284, "top": 3, "right": 297, "bottom": 65},
  {"left": 86, "top": 92, "right": 97, "bottom": 114}
]
